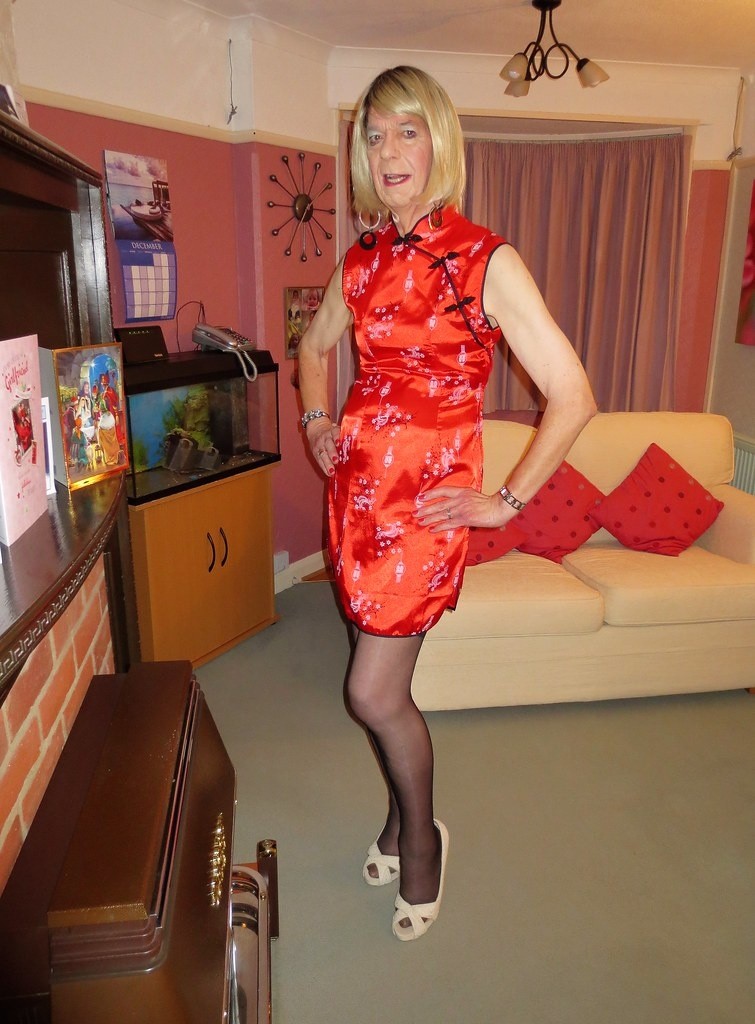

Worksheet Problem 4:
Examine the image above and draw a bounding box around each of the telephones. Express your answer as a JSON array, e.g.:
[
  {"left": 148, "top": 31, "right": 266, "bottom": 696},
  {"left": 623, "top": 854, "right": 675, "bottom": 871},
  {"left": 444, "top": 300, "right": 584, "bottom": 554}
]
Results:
[{"left": 191, "top": 322, "right": 256, "bottom": 352}]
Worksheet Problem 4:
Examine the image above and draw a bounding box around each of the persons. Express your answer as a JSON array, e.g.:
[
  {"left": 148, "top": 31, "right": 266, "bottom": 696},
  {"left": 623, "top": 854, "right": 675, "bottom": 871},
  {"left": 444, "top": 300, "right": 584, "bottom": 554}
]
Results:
[{"left": 294, "top": 64, "right": 598, "bottom": 941}]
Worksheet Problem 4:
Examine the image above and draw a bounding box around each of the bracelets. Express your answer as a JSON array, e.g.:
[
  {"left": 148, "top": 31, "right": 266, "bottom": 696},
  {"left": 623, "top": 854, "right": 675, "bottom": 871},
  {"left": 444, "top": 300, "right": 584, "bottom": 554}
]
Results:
[{"left": 301, "top": 409, "right": 330, "bottom": 430}]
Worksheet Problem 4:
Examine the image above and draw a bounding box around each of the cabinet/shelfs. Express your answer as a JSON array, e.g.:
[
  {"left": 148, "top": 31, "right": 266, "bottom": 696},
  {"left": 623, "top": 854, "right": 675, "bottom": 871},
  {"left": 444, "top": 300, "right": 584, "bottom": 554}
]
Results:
[{"left": 128, "top": 465, "right": 279, "bottom": 671}]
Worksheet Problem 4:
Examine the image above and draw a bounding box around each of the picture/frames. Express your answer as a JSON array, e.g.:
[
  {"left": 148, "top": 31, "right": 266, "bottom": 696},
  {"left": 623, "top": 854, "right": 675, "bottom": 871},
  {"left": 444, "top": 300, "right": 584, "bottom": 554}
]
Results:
[{"left": 284, "top": 287, "right": 325, "bottom": 358}]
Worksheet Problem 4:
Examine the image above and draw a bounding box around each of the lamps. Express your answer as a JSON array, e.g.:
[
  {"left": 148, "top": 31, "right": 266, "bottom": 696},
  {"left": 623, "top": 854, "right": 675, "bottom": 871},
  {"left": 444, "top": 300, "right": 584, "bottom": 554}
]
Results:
[{"left": 499, "top": 0, "right": 611, "bottom": 97}]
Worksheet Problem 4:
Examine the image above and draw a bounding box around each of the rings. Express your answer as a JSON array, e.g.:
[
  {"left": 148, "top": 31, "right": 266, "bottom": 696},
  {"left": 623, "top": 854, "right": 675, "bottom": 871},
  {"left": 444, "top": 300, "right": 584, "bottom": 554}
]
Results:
[{"left": 448, "top": 508, "right": 451, "bottom": 520}]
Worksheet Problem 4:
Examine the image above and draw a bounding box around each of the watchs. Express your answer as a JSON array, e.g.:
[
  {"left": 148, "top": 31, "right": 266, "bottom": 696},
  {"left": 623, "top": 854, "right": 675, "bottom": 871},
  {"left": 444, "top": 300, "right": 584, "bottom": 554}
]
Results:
[{"left": 499, "top": 485, "right": 527, "bottom": 510}]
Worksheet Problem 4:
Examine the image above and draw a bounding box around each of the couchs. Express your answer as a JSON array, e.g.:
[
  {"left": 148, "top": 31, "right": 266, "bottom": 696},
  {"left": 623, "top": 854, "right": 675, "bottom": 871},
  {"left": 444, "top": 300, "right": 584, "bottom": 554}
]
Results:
[{"left": 410, "top": 412, "right": 755, "bottom": 712}]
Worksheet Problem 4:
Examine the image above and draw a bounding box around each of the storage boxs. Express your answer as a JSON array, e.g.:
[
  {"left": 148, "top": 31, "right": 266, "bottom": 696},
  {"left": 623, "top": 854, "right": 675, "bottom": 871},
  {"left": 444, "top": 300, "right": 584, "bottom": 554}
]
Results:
[{"left": 122, "top": 347, "right": 280, "bottom": 507}]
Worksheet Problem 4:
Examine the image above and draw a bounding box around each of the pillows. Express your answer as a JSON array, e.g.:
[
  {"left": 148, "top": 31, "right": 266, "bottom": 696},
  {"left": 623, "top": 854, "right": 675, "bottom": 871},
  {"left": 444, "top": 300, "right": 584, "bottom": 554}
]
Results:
[
  {"left": 463, "top": 504, "right": 529, "bottom": 567},
  {"left": 588, "top": 442, "right": 725, "bottom": 558},
  {"left": 507, "top": 461, "right": 606, "bottom": 564}
]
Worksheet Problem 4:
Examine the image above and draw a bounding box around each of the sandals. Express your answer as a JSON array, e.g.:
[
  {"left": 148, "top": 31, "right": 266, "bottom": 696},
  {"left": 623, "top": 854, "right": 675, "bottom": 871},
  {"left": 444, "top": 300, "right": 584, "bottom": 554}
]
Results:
[
  {"left": 392, "top": 818, "right": 449, "bottom": 941},
  {"left": 363, "top": 820, "right": 400, "bottom": 886}
]
